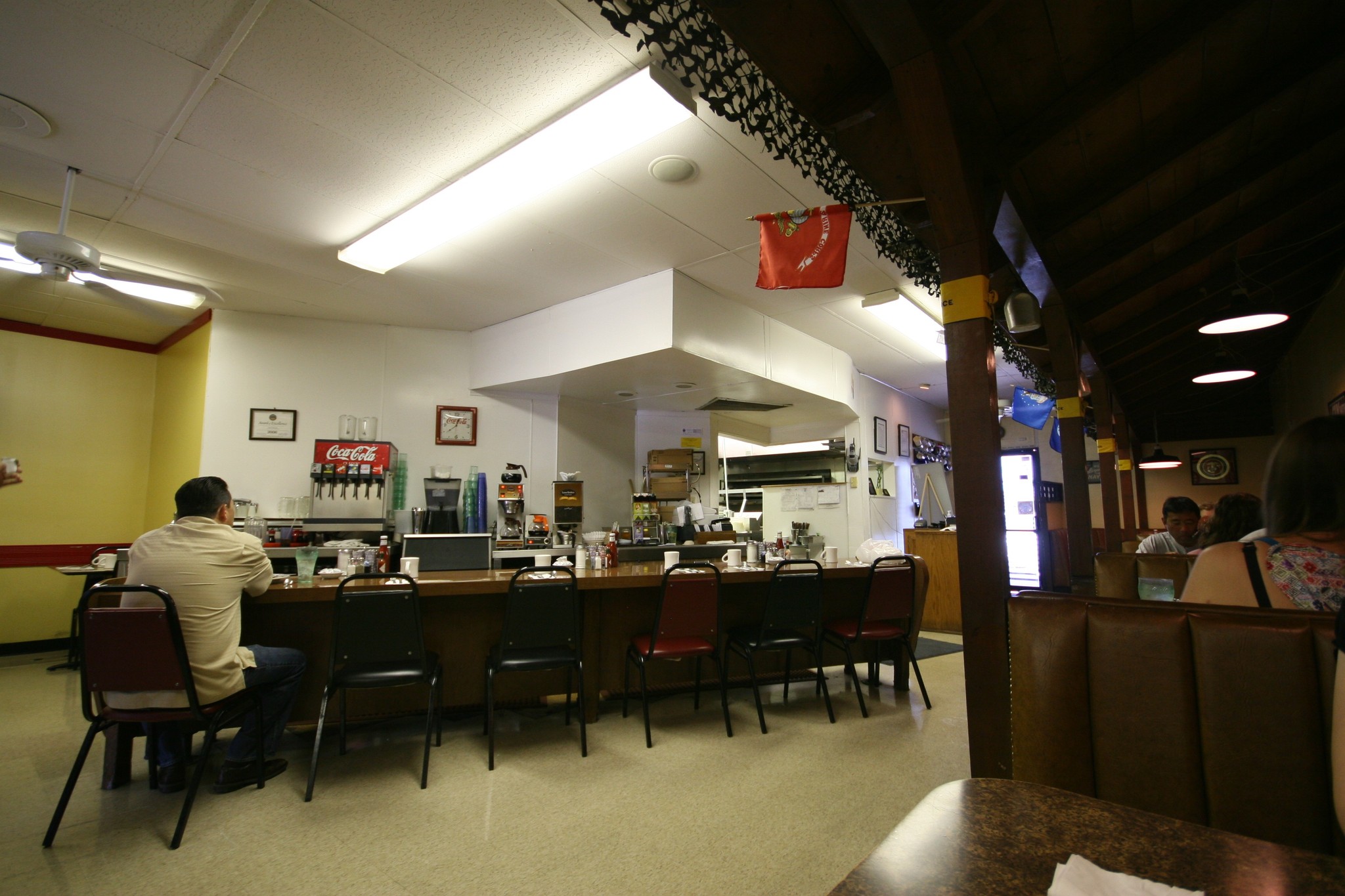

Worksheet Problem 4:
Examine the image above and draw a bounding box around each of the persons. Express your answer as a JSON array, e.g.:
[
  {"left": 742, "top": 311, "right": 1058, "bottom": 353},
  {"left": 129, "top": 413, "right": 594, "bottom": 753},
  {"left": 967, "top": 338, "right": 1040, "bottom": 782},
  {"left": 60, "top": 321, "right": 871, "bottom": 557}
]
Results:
[
  {"left": 1135, "top": 496, "right": 1201, "bottom": 554},
  {"left": 1179, "top": 413, "right": 1345, "bottom": 611},
  {"left": 1198, "top": 502, "right": 1216, "bottom": 517},
  {"left": 118, "top": 476, "right": 308, "bottom": 795},
  {"left": 1188, "top": 492, "right": 1264, "bottom": 554}
]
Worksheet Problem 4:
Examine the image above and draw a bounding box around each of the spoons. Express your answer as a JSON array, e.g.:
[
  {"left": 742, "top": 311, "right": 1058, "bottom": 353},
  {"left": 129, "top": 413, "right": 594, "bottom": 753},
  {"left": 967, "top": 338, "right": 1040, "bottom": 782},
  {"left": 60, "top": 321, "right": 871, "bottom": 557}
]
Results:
[{"left": 552, "top": 571, "right": 557, "bottom": 578}]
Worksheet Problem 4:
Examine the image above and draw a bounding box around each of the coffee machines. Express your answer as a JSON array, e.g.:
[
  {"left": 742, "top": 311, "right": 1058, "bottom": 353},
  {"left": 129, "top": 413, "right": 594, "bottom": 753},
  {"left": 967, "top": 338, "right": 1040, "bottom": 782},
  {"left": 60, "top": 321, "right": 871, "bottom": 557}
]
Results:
[
  {"left": 497, "top": 483, "right": 525, "bottom": 550},
  {"left": 551, "top": 480, "right": 584, "bottom": 549}
]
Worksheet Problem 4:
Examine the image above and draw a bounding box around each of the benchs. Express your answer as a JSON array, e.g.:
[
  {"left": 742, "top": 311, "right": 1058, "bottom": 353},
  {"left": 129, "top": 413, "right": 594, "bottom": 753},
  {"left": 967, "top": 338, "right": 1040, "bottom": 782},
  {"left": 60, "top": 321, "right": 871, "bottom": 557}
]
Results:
[
  {"left": 1007, "top": 589, "right": 1344, "bottom": 859},
  {"left": 1052, "top": 523, "right": 1167, "bottom": 589},
  {"left": 1095, "top": 554, "right": 1200, "bottom": 600}
]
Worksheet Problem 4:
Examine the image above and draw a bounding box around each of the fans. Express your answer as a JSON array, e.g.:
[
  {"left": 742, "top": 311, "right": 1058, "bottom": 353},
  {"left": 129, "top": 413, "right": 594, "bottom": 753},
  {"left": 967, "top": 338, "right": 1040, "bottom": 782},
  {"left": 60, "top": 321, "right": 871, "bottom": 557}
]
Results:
[{"left": 0, "top": 167, "right": 223, "bottom": 319}]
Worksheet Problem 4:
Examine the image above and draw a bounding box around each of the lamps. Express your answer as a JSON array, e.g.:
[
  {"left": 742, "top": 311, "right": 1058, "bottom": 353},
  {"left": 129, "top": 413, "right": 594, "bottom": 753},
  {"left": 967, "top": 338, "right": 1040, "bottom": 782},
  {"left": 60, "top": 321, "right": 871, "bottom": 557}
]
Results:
[
  {"left": 862, "top": 289, "right": 946, "bottom": 361},
  {"left": 1004, "top": 263, "right": 1040, "bottom": 333},
  {"left": 1077, "top": 370, "right": 1092, "bottom": 397},
  {"left": 337, "top": 62, "right": 698, "bottom": 274},
  {"left": 1138, "top": 369, "right": 1183, "bottom": 470},
  {"left": 1197, "top": 239, "right": 1290, "bottom": 335},
  {"left": 1192, "top": 335, "right": 1258, "bottom": 383}
]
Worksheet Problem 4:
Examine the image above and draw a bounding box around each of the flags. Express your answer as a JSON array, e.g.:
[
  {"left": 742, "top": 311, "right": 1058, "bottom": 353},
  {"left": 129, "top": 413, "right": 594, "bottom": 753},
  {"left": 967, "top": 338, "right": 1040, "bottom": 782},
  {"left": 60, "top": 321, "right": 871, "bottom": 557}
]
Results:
[
  {"left": 1049, "top": 416, "right": 1088, "bottom": 453},
  {"left": 1011, "top": 385, "right": 1056, "bottom": 430},
  {"left": 755, "top": 203, "right": 852, "bottom": 289}
]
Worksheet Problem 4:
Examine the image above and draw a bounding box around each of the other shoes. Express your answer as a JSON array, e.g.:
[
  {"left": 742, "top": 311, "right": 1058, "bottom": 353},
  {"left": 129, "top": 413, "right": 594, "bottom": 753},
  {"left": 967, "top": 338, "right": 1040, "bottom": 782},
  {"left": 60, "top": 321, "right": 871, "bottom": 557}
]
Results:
[
  {"left": 213, "top": 758, "right": 288, "bottom": 795},
  {"left": 159, "top": 758, "right": 196, "bottom": 792}
]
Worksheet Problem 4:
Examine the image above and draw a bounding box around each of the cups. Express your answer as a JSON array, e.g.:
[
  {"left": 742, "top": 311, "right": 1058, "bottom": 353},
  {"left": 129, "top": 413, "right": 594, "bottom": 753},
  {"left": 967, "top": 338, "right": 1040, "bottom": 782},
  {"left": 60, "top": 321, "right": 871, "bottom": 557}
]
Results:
[
  {"left": 389, "top": 454, "right": 407, "bottom": 520},
  {"left": 295, "top": 547, "right": 318, "bottom": 584},
  {"left": 791, "top": 529, "right": 809, "bottom": 544},
  {"left": 913, "top": 435, "right": 952, "bottom": 471},
  {"left": 280, "top": 527, "right": 293, "bottom": 547},
  {"left": 399, "top": 557, "right": 420, "bottom": 577},
  {"left": 722, "top": 549, "right": 741, "bottom": 566},
  {"left": 463, "top": 467, "right": 488, "bottom": 534},
  {"left": 91, "top": 553, "right": 117, "bottom": 569},
  {"left": 664, "top": 551, "right": 680, "bottom": 570},
  {"left": 277, "top": 496, "right": 310, "bottom": 517},
  {"left": 820, "top": 546, "right": 838, "bottom": 563}
]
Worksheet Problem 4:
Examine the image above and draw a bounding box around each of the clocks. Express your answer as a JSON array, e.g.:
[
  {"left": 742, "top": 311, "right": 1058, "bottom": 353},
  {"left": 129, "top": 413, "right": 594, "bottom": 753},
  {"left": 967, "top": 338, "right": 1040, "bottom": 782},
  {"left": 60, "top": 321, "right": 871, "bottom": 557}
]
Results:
[{"left": 436, "top": 405, "right": 477, "bottom": 446}]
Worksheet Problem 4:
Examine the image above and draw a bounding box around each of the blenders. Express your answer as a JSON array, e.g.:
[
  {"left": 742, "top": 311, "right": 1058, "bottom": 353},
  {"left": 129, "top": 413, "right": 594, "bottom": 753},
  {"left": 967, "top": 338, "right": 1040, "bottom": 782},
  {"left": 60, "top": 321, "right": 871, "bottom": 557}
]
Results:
[{"left": 421, "top": 478, "right": 461, "bottom": 534}]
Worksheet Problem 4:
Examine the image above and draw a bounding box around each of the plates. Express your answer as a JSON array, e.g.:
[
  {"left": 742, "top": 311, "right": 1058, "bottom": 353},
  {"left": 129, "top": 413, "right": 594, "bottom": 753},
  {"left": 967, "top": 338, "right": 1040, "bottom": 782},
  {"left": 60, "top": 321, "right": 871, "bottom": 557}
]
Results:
[
  {"left": 317, "top": 572, "right": 344, "bottom": 578},
  {"left": 617, "top": 538, "right": 632, "bottom": 544},
  {"left": 271, "top": 574, "right": 289, "bottom": 584},
  {"left": 553, "top": 562, "right": 573, "bottom": 568},
  {"left": 767, "top": 560, "right": 786, "bottom": 565}
]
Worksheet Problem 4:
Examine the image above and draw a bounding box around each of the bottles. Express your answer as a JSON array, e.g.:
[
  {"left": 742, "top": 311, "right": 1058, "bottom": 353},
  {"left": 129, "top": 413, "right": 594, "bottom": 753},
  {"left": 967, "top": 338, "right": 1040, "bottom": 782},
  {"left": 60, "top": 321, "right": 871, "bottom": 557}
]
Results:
[
  {"left": 576, "top": 522, "right": 620, "bottom": 570},
  {"left": 746, "top": 531, "right": 791, "bottom": 563},
  {"left": 336, "top": 535, "right": 389, "bottom": 576},
  {"left": 268, "top": 530, "right": 275, "bottom": 543}
]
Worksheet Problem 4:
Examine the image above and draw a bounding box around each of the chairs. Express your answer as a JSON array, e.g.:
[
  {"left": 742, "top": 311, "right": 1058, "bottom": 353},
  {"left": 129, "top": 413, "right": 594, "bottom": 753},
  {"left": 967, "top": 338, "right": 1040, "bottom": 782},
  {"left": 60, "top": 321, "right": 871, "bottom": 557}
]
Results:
[
  {"left": 624, "top": 563, "right": 735, "bottom": 749},
  {"left": 481, "top": 570, "right": 593, "bottom": 769},
  {"left": 721, "top": 559, "right": 837, "bottom": 734},
  {"left": 817, "top": 557, "right": 933, "bottom": 716},
  {"left": 43, "top": 581, "right": 275, "bottom": 849},
  {"left": 304, "top": 571, "right": 449, "bottom": 803}
]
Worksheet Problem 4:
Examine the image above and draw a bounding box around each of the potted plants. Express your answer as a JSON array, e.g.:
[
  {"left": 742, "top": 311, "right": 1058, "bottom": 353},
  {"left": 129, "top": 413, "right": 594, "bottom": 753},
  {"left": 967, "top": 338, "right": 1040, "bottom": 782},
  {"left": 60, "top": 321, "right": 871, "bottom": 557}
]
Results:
[{"left": 875, "top": 463, "right": 884, "bottom": 495}]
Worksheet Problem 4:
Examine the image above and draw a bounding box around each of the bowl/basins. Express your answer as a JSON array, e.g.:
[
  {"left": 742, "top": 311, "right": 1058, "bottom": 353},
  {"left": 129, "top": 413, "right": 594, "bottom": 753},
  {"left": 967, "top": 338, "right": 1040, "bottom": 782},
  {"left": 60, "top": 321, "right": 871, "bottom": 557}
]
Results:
[{"left": 429, "top": 465, "right": 453, "bottom": 478}]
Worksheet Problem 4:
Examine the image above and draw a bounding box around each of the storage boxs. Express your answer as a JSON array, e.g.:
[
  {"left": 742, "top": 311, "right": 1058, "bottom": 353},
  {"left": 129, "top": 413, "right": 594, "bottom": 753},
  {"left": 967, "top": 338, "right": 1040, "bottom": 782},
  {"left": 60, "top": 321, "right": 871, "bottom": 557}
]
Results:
[
  {"left": 657, "top": 505, "right": 677, "bottom": 523},
  {"left": 648, "top": 449, "right": 694, "bottom": 472},
  {"left": 646, "top": 477, "right": 689, "bottom": 500}
]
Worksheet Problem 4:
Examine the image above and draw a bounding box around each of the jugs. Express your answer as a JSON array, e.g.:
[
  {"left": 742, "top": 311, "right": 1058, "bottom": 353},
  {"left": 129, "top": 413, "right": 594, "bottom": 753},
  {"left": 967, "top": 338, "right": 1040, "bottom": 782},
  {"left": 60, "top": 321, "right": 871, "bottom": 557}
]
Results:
[
  {"left": 338, "top": 414, "right": 378, "bottom": 441},
  {"left": 244, "top": 516, "right": 267, "bottom": 548},
  {"left": 498, "top": 518, "right": 523, "bottom": 539},
  {"left": 230, "top": 499, "right": 253, "bottom": 517},
  {"left": 501, "top": 463, "right": 528, "bottom": 484},
  {"left": 528, "top": 514, "right": 549, "bottom": 536}
]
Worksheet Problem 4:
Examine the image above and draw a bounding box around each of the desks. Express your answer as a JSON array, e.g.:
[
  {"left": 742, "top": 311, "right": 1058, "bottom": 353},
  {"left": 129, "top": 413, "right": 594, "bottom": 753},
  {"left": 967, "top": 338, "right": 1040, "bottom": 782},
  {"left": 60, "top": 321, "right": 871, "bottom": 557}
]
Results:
[{"left": 822, "top": 779, "right": 1344, "bottom": 896}]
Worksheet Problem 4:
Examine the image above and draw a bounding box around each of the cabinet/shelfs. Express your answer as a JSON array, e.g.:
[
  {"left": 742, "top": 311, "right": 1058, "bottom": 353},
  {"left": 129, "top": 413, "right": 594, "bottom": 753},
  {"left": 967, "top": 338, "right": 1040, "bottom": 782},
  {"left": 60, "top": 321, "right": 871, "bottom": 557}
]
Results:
[{"left": 642, "top": 464, "right": 691, "bottom": 525}]
